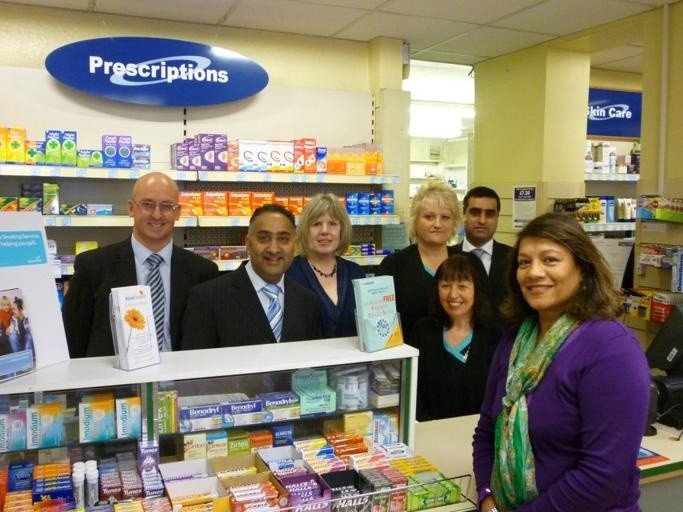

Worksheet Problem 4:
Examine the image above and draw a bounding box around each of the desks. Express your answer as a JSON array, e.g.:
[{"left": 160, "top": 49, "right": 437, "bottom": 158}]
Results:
[{"left": 415, "top": 413, "right": 683, "bottom": 512}]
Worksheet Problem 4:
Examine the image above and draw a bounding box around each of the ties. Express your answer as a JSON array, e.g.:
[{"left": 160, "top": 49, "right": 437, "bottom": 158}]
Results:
[
  {"left": 262, "top": 284, "right": 283, "bottom": 342},
  {"left": 146, "top": 254, "right": 166, "bottom": 350},
  {"left": 473, "top": 247, "right": 484, "bottom": 260}
]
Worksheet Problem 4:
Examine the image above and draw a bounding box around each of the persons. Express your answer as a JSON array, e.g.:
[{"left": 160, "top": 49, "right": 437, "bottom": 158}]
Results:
[
  {"left": 374, "top": 185, "right": 462, "bottom": 341},
  {"left": 0, "top": 296, "right": 31, "bottom": 356},
  {"left": 407, "top": 253, "right": 511, "bottom": 422},
  {"left": 472, "top": 213, "right": 651, "bottom": 512},
  {"left": 451, "top": 186, "right": 513, "bottom": 320},
  {"left": 286, "top": 194, "right": 366, "bottom": 339},
  {"left": 60, "top": 172, "right": 219, "bottom": 359},
  {"left": 179, "top": 202, "right": 324, "bottom": 351}
]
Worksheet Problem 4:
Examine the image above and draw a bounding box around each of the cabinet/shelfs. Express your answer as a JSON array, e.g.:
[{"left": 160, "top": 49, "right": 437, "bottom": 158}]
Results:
[
  {"left": 0, "top": 164, "right": 401, "bottom": 275},
  {"left": 584, "top": 173, "right": 640, "bottom": 232}
]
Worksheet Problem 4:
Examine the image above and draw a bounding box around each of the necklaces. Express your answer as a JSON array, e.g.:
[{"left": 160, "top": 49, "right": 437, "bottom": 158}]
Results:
[{"left": 313, "top": 264, "right": 337, "bottom": 278}]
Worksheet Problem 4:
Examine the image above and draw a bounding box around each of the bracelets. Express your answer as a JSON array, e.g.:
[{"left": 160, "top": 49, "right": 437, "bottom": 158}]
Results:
[{"left": 488, "top": 508, "right": 498, "bottom": 512}]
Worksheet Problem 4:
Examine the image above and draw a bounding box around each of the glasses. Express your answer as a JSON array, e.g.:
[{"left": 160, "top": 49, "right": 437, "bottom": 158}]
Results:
[{"left": 132, "top": 199, "right": 178, "bottom": 212}]
